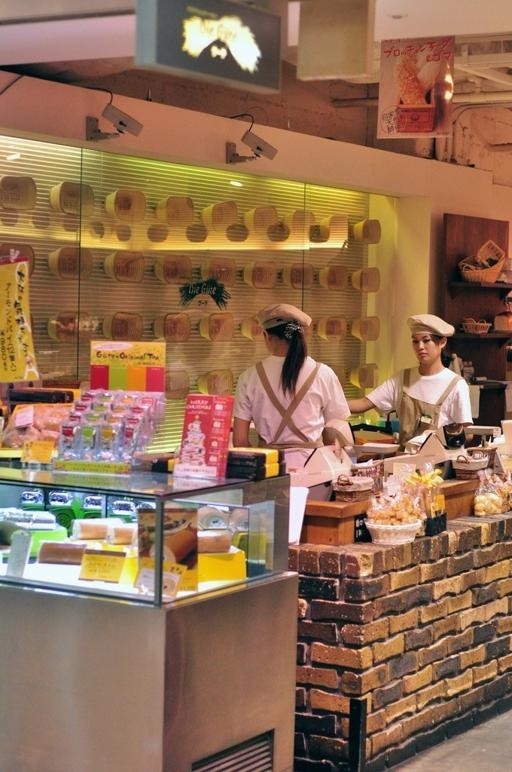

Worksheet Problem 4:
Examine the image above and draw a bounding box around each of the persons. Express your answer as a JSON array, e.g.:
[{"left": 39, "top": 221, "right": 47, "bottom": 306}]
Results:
[
  {"left": 233, "top": 305, "right": 350, "bottom": 501},
  {"left": 346, "top": 315, "right": 472, "bottom": 449}
]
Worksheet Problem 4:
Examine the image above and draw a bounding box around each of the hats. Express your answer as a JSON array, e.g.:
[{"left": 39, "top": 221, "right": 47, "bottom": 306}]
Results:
[
  {"left": 254, "top": 303, "right": 312, "bottom": 329},
  {"left": 406, "top": 314, "right": 455, "bottom": 337}
]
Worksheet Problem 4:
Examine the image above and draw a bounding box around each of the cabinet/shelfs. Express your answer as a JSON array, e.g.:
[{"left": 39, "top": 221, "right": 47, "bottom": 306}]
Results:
[
  {"left": 435, "top": 211, "right": 512, "bottom": 426},
  {"left": 290, "top": 479, "right": 479, "bottom": 548},
  {"left": 0, "top": 459, "right": 299, "bottom": 772}
]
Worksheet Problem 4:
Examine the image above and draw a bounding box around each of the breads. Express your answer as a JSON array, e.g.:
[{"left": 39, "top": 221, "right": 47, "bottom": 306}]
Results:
[
  {"left": 38, "top": 543, "right": 86, "bottom": 565},
  {"left": 198, "top": 506, "right": 229, "bottom": 531},
  {"left": 230, "top": 509, "right": 248, "bottom": 533},
  {"left": 73, "top": 518, "right": 123, "bottom": 539},
  {"left": 198, "top": 530, "right": 231, "bottom": 553},
  {"left": 113, "top": 525, "right": 139, "bottom": 544},
  {"left": 148, "top": 526, "right": 197, "bottom": 565},
  {"left": 366, "top": 497, "right": 418, "bottom": 527},
  {"left": 410, "top": 485, "right": 446, "bottom": 517},
  {"left": 475, "top": 493, "right": 504, "bottom": 516}
]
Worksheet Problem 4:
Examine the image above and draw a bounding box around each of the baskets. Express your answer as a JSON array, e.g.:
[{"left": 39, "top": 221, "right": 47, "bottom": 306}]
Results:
[
  {"left": 331, "top": 473, "right": 374, "bottom": 504},
  {"left": 451, "top": 452, "right": 488, "bottom": 480},
  {"left": 363, "top": 519, "right": 421, "bottom": 545},
  {"left": 468, "top": 446, "right": 497, "bottom": 466}
]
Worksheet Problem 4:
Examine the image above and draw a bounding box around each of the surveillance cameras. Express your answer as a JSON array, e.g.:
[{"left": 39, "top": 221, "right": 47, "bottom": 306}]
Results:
[
  {"left": 85, "top": 103, "right": 144, "bottom": 142},
  {"left": 225, "top": 130, "right": 279, "bottom": 164}
]
